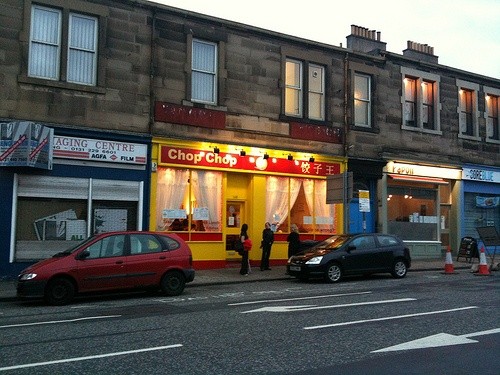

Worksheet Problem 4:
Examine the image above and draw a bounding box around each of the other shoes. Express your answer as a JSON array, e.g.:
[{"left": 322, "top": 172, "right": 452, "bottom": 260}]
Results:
[
  {"left": 261, "top": 268, "right": 264, "bottom": 271},
  {"left": 265, "top": 267, "right": 272, "bottom": 270},
  {"left": 285, "top": 272, "right": 290, "bottom": 275},
  {"left": 241, "top": 272, "right": 249, "bottom": 276}
]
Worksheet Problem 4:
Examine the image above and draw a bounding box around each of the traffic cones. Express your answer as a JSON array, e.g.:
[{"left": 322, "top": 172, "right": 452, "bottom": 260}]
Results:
[
  {"left": 473, "top": 246, "right": 496, "bottom": 276},
  {"left": 441, "top": 243, "right": 460, "bottom": 274}
]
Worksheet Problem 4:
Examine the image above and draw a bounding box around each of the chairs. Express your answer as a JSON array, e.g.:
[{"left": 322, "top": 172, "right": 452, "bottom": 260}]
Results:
[
  {"left": 360, "top": 238, "right": 390, "bottom": 249},
  {"left": 114, "top": 241, "right": 124, "bottom": 258}
]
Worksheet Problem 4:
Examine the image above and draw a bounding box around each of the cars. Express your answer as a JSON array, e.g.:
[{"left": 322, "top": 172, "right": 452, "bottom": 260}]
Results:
[
  {"left": 16, "top": 230, "right": 195, "bottom": 306},
  {"left": 286, "top": 232, "right": 412, "bottom": 284}
]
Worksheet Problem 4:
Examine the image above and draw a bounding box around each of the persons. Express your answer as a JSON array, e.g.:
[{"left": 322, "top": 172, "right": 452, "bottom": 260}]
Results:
[
  {"left": 260, "top": 222, "right": 274, "bottom": 270},
  {"left": 239, "top": 224, "right": 252, "bottom": 276},
  {"left": 285, "top": 224, "right": 300, "bottom": 275}
]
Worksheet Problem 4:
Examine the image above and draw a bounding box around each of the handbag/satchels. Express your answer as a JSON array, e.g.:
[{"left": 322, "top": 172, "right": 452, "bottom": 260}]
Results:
[{"left": 234, "top": 235, "right": 242, "bottom": 251}]
